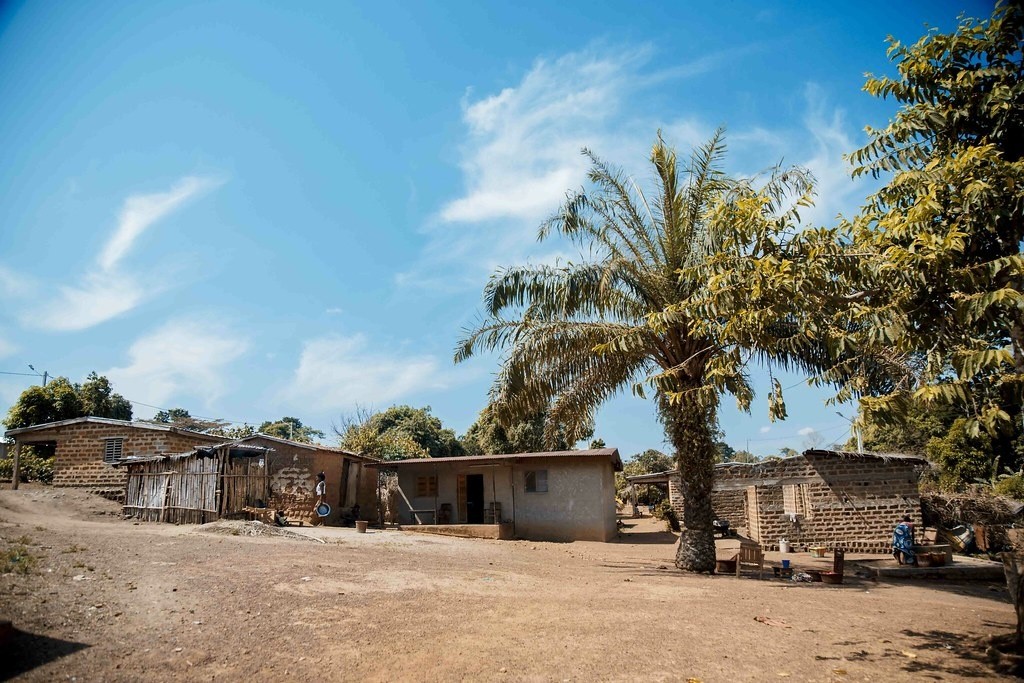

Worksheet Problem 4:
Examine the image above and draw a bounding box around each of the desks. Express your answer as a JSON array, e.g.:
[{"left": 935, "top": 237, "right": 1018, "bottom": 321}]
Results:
[
  {"left": 912, "top": 545, "right": 953, "bottom": 564},
  {"left": 772, "top": 566, "right": 793, "bottom": 578},
  {"left": 410, "top": 510, "right": 436, "bottom": 525}
]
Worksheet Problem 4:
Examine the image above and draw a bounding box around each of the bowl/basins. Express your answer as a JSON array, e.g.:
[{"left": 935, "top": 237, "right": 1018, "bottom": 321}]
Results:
[
  {"left": 316, "top": 503, "right": 331, "bottom": 518},
  {"left": 806, "top": 570, "right": 828, "bottom": 581},
  {"left": 916, "top": 552, "right": 946, "bottom": 567},
  {"left": 820, "top": 572, "right": 843, "bottom": 583}
]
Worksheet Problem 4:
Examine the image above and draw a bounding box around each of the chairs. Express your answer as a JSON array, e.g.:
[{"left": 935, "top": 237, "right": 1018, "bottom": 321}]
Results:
[
  {"left": 736, "top": 542, "right": 766, "bottom": 580},
  {"left": 483, "top": 502, "right": 501, "bottom": 524},
  {"left": 433, "top": 503, "right": 452, "bottom": 524}
]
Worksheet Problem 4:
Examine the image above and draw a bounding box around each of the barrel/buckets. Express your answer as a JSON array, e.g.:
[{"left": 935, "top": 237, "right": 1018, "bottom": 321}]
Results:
[
  {"left": 779, "top": 540, "right": 790, "bottom": 554},
  {"left": 355, "top": 521, "right": 368, "bottom": 533}
]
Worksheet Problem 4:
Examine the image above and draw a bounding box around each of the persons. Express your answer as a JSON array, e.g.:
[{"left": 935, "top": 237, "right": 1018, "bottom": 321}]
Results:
[
  {"left": 892, "top": 516, "right": 915, "bottom": 565},
  {"left": 634, "top": 497, "right": 661, "bottom": 518},
  {"left": 313, "top": 473, "right": 326, "bottom": 527}
]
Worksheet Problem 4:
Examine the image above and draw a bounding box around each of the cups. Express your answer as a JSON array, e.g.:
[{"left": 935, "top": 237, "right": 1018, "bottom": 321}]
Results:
[{"left": 782, "top": 560, "right": 790, "bottom": 568}]
